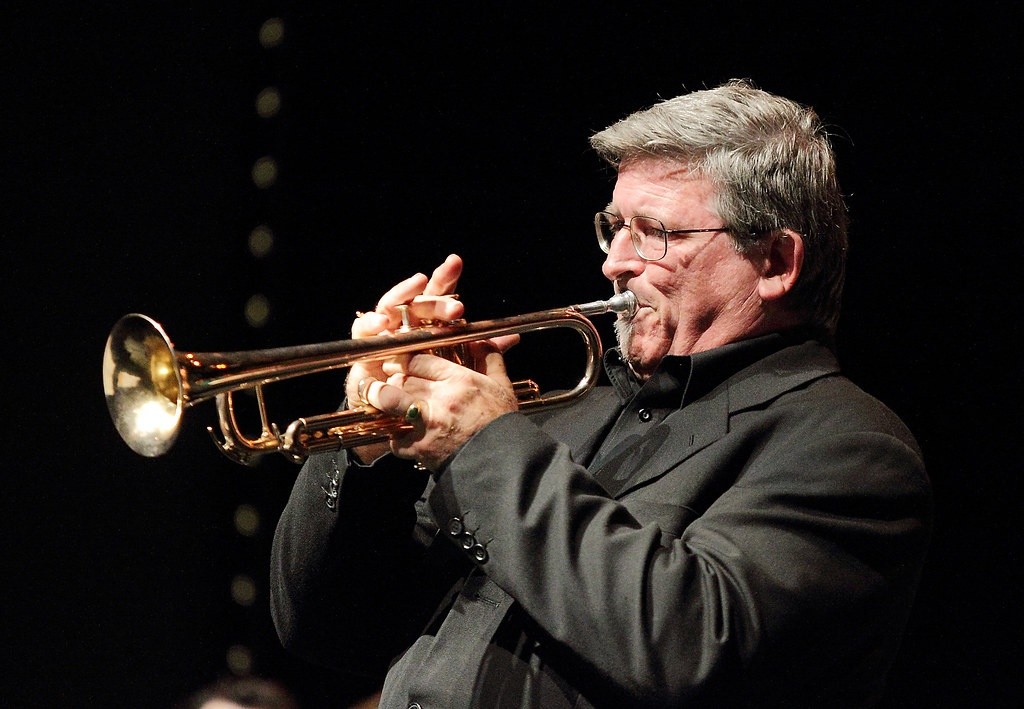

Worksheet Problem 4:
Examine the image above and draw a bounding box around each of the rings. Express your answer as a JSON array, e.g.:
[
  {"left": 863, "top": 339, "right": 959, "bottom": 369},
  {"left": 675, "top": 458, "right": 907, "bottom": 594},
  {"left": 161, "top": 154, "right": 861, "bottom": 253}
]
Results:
[
  {"left": 405, "top": 400, "right": 421, "bottom": 420},
  {"left": 357, "top": 374, "right": 377, "bottom": 404}
]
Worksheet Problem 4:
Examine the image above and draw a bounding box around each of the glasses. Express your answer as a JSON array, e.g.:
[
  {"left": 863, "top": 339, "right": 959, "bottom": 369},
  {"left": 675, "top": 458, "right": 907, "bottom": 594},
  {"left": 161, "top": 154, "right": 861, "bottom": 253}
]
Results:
[{"left": 594, "top": 210, "right": 733, "bottom": 261}]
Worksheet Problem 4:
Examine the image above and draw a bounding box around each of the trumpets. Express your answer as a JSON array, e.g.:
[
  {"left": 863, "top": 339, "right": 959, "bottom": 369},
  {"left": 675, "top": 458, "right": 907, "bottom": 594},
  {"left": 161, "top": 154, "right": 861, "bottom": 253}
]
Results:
[{"left": 99, "top": 287, "right": 642, "bottom": 468}]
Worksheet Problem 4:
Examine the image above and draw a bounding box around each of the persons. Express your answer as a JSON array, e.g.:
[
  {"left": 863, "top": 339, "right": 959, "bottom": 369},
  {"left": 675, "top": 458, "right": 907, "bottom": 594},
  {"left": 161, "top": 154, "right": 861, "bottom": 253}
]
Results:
[
  {"left": 199, "top": 682, "right": 291, "bottom": 709},
  {"left": 270, "top": 77, "right": 929, "bottom": 709}
]
